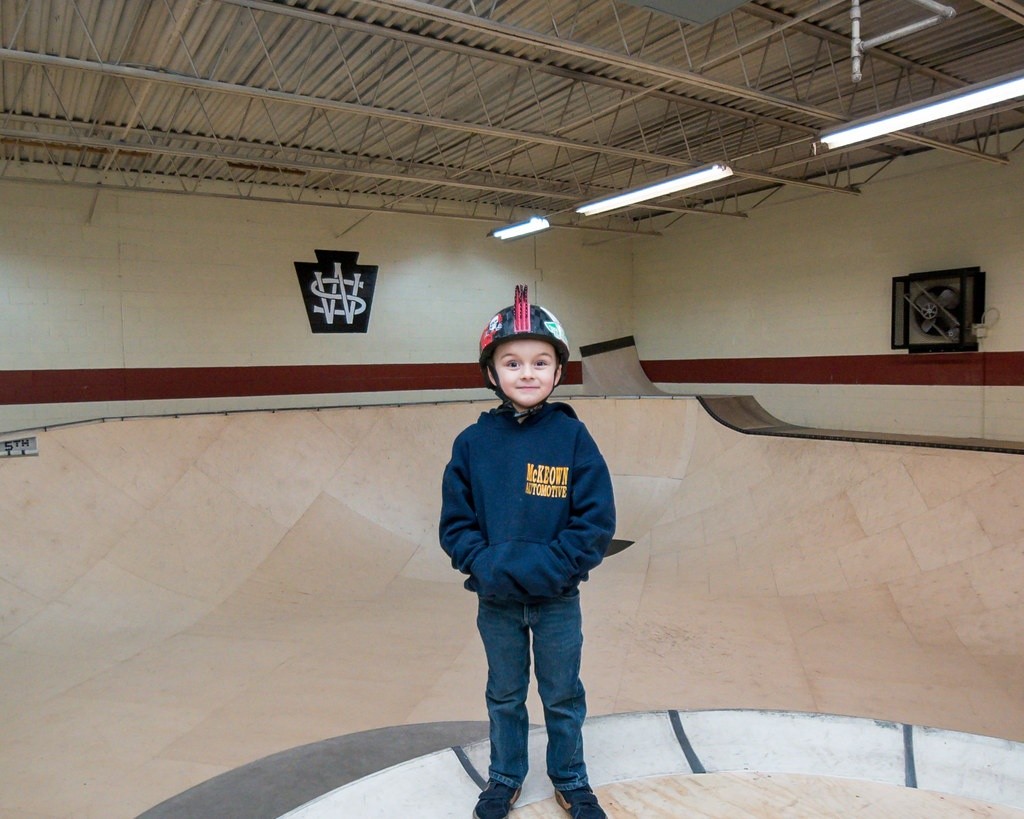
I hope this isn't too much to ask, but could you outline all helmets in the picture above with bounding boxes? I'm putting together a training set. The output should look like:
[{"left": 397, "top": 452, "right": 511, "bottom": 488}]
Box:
[{"left": 476, "top": 303, "right": 571, "bottom": 391}]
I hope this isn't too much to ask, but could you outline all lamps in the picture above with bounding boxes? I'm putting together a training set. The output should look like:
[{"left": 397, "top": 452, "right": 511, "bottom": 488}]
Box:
[
  {"left": 573, "top": 160, "right": 735, "bottom": 216},
  {"left": 484, "top": 213, "right": 551, "bottom": 242},
  {"left": 816, "top": 69, "right": 1024, "bottom": 153}
]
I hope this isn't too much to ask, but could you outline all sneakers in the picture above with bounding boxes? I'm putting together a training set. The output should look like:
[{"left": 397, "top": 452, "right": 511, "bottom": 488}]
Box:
[
  {"left": 471, "top": 778, "right": 523, "bottom": 819},
  {"left": 554, "top": 783, "right": 609, "bottom": 819}
]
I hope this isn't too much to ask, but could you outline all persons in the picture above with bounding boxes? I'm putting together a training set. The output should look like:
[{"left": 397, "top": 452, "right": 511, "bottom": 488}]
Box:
[{"left": 439, "top": 282, "right": 616, "bottom": 819}]
